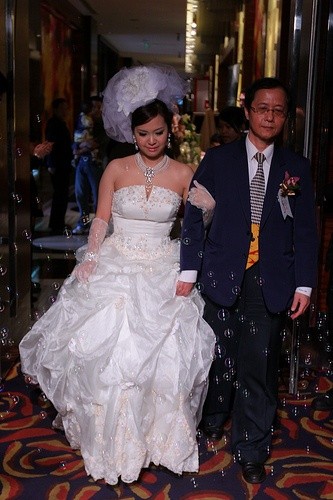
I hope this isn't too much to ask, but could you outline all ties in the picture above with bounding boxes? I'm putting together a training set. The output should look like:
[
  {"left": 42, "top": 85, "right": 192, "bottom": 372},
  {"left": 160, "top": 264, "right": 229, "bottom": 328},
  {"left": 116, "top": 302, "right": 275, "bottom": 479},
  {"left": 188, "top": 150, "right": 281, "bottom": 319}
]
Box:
[{"left": 245, "top": 153, "right": 265, "bottom": 269}]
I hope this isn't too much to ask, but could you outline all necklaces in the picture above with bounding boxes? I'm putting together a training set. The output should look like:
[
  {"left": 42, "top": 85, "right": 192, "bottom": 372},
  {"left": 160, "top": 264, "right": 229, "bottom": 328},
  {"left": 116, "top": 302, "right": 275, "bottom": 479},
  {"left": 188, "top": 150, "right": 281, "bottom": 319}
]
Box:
[{"left": 135, "top": 151, "right": 170, "bottom": 183}]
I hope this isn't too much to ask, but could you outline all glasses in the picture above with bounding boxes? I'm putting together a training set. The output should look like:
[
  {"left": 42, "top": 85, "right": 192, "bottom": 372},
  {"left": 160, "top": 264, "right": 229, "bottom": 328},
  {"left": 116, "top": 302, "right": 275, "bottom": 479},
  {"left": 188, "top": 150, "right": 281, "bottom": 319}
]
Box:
[{"left": 248, "top": 104, "right": 287, "bottom": 117}]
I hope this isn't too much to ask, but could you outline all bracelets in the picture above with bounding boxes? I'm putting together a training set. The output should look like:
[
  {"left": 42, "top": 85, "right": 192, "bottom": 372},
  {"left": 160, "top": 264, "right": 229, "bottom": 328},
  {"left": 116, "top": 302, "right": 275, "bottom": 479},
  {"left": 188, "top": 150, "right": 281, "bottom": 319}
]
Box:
[{"left": 83, "top": 252, "right": 98, "bottom": 262}]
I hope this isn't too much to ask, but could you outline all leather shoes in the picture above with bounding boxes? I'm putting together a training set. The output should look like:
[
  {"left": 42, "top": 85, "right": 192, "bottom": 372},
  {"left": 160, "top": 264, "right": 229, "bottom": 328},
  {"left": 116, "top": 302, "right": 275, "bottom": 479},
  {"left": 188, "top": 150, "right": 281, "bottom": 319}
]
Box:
[
  {"left": 242, "top": 461, "right": 266, "bottom": 483},
  {"left": 204, "top": 418, "right": 225, "bottom": 438}
]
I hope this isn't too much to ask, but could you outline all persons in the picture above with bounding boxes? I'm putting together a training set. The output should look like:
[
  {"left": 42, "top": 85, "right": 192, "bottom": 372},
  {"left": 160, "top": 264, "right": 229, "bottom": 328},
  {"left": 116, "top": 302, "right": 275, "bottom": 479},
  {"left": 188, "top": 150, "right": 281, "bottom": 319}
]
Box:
[
  {"left": 17, "top": 66, "right": 217, "bottom": 486},
  {"left": 176, "top": 77, "right": 321, "bottom": 483},
  {"left": 44, "top": 95, "right": 245, "bottom": 234}
]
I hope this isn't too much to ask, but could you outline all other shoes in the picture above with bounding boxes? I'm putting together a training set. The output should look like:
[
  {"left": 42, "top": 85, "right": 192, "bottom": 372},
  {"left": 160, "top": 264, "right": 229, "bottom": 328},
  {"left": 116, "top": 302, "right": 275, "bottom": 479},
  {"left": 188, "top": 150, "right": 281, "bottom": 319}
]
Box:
[{"left": 72, "top": 225, "right": 90, "bottom": 234}]
